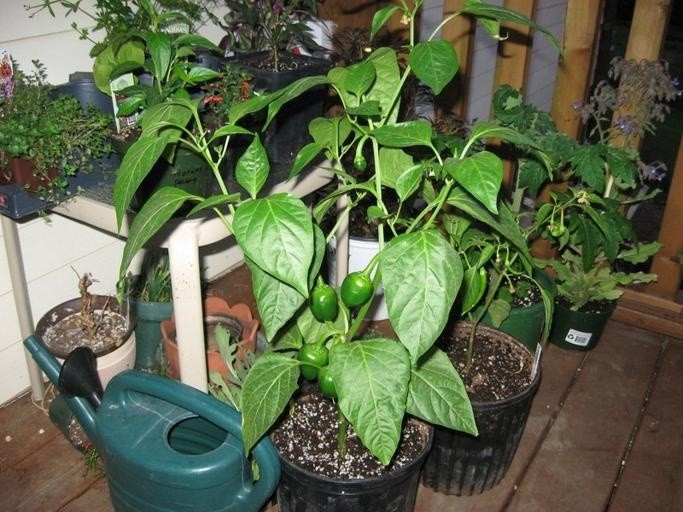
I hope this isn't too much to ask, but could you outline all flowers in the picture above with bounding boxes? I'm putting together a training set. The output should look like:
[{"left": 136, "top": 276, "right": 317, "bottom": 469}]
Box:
[
  {"left": 0, "top": 49, "right": 119, "bottom": 229},
  {"left": 572, "top": 56, "right": 683, "bottom": 185}
]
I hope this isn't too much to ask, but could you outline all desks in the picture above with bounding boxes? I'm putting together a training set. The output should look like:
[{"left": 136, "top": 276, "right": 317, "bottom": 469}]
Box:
[{"left": 61, "top": 150, "right": 351, "bottom": 396}]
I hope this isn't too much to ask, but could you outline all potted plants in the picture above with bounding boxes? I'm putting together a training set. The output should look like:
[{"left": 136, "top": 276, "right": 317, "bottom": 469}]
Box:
[
  {"left": 468, "top": 86, "right": 640, "bottom": 346},
  {"left": 549, "top": 169, "right": 661, "bottom": 351},
  {"left": 40, "top": 3, "right": 332, "bottom": 162},
  {"left": 116, "top": 261, "right": 170, "bottom": 366},
  {"left": 113, "top": 5, "right": 567, "bottom": 510},
  {"left": 418, "top": 151, "right": 600, "bottom": 495},
  {"left": 36, "top": 270, "right": 135, "bottom": 389}
]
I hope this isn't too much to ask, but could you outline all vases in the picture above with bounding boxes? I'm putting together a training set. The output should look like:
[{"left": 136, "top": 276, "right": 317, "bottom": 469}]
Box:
[
  {"left": 164, "top": 298, "right": 259, "bottom": 383},
  {"left": 3, "top": 153, "right": 60, "bottom": 191}
]
